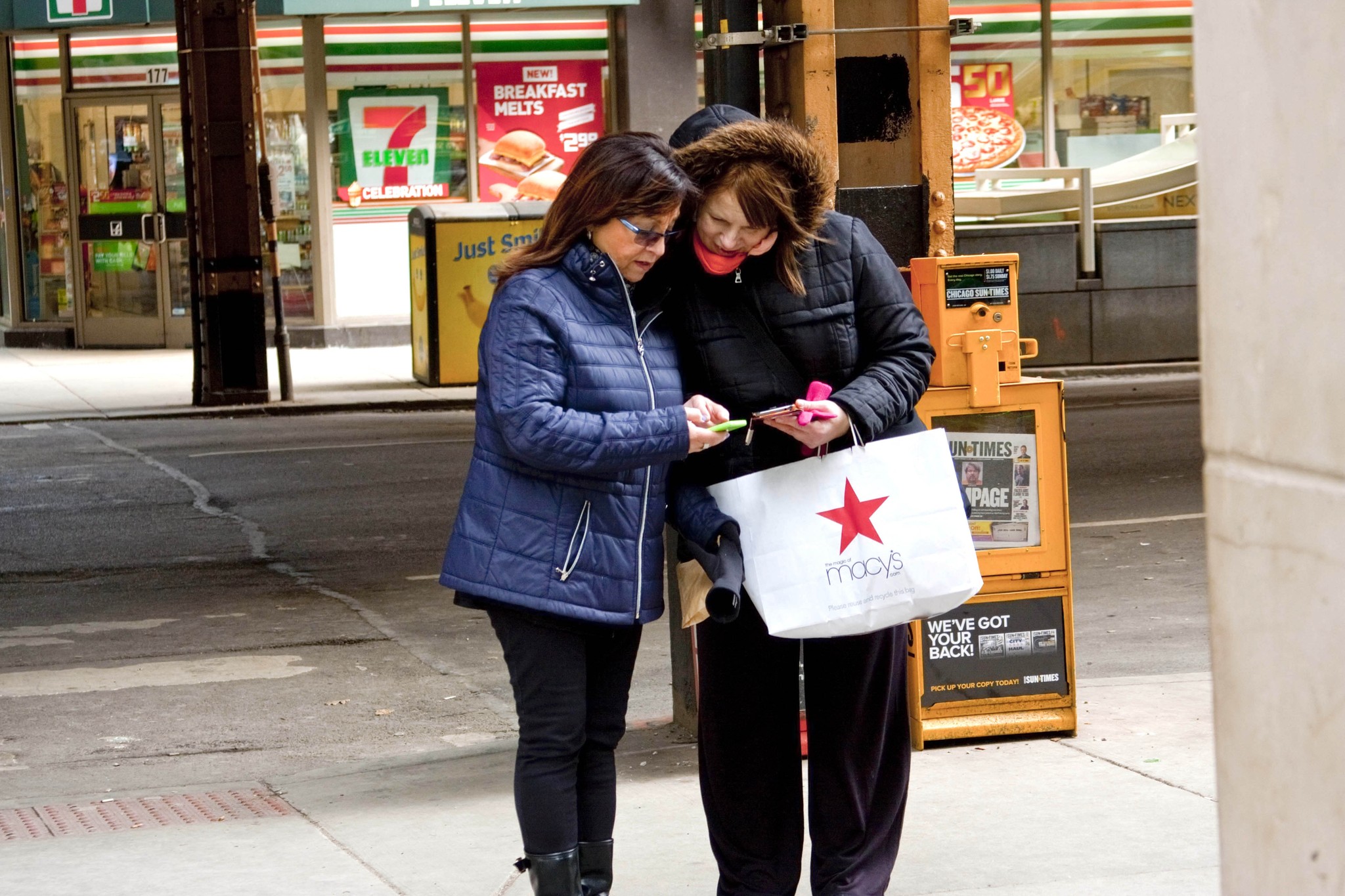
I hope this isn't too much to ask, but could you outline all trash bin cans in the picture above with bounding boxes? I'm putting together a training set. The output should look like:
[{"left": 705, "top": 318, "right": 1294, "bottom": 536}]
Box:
[{"left": 409, "top": 200, "right": 561, "bottom": 388}]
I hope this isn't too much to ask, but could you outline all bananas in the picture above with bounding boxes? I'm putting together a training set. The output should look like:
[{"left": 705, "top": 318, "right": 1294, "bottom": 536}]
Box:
[{"left": 463, "top": 285, "right": 490, "bottom": 328}]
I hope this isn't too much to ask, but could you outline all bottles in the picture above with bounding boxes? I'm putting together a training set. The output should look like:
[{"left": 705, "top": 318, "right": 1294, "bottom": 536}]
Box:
[{"left": 259, "top": 113, "right": 313, "bottom": 252}]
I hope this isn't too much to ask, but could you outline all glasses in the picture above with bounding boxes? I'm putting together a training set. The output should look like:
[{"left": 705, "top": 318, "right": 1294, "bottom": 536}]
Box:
[{"left": 617, "top": 217, "right": 681, "bottom": 247}]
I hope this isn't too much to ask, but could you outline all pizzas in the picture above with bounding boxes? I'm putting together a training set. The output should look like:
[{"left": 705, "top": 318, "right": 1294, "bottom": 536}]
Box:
[{"left": 950, "top": 105, "right": 1022, "bottom": 174}]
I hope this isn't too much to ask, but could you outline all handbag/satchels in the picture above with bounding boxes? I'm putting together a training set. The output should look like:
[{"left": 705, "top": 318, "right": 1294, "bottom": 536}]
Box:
[{"left": 708, "top": 402, "right": 984, "bottom": 639}]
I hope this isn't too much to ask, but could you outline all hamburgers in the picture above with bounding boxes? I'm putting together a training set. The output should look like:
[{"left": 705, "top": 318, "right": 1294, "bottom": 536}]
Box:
[
  {"left": 513, "top": 169, "right": 567, "bottom": 201},
  {"left": 490, "top": 129, "right": 548, "bottom": 172}
]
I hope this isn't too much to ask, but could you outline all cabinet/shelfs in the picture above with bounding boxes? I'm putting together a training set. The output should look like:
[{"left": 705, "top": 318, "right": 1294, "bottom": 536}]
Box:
[{"left": 27, "top": 104, "right": 478, "bottom": 319}]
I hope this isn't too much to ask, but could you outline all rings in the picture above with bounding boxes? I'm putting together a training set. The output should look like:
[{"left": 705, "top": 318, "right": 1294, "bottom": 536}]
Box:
[{"left": 701, "top": 443, "right": 710, "bottom": 449}]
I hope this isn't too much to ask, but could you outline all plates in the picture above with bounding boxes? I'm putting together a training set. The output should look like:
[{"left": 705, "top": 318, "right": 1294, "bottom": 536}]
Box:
[
  {"left": 478, "top": 146, "right": 555, "bottom": 176},
  {"left": 952, "top": 118, "right": 1027, "bottom": 177}
]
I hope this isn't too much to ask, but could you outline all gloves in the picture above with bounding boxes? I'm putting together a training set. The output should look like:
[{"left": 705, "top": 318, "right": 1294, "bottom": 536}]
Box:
[{"left": 686, "top": 518, "right": 745, "bottom": 622}]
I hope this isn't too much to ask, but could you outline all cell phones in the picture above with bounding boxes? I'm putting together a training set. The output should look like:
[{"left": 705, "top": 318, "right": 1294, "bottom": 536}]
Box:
[
  {"left": 708, "top": 419, "right": 747, "bottom": 432},
  {"left": 752, "top": 405, "right": 797, "bottom": 418}
]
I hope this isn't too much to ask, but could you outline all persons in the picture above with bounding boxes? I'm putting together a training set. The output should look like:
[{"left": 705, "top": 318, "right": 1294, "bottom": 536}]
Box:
[
  {"left": 962, "top": 462, "right": 982, "bottom": 484},
  {"left": 647, "top": 103, "right": 972, "bottom": 896},
  {"left": 436, "top": 132, "right": 730, "bottom": 896},
  {"left": 1020, "top": 500, "right": 1028, "bottom": 509},
  {"left": 1017, "top": 445, "right": 1030, "bottom": 458},
  {"left": 1015, "top": 465, "right": 1029, "bottom": 486}
]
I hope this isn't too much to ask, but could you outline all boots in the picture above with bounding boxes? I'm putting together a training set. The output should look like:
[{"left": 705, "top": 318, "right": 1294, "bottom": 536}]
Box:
[
  {"left": 513, "top": 843, "right": 582, "bottom": 896},
  {"left": 577, "top": 838, "right": 614, "bottom": 896}
]
